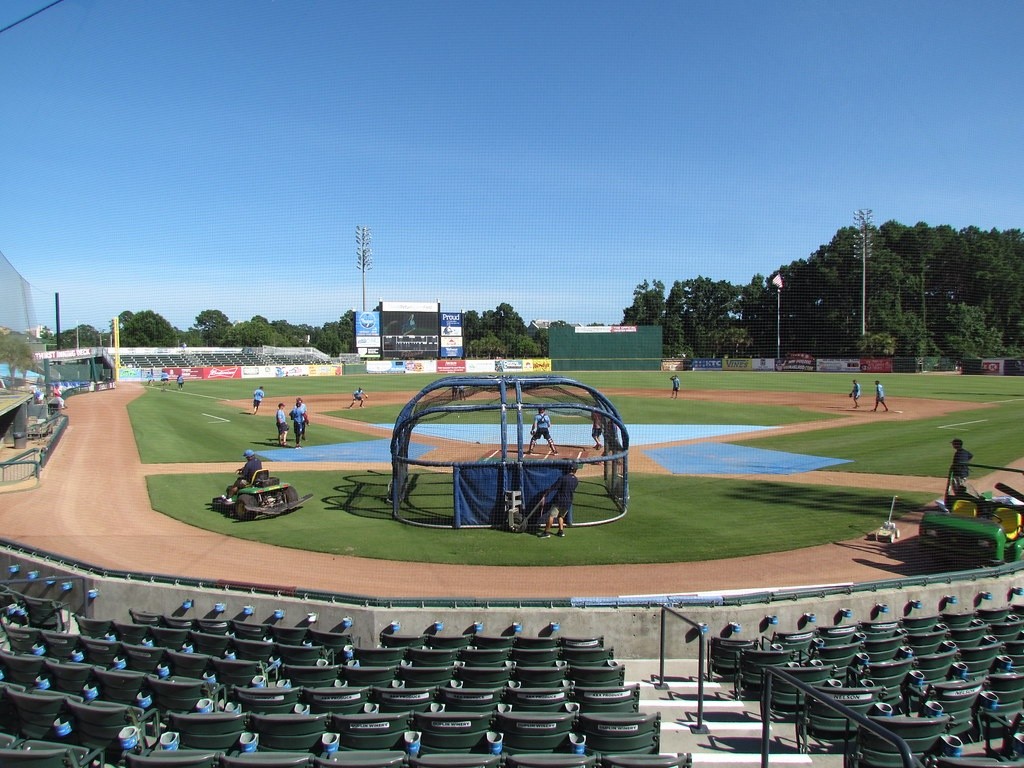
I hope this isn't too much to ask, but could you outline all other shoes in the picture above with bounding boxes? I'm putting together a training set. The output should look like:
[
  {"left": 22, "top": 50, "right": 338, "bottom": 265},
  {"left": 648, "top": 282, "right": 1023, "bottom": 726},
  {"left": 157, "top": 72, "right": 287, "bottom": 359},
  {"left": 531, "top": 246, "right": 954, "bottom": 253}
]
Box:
[
  {"left": 224, "top": 498, "right": 232, "bottom": 503},
  {"left": 295, "top": 446, "right": 302, "bottom": 449},
  {"left": 593, "top": 444, "right": 603, "bottom": 450},
  {"left": 554, "top": 451, "right": 560, "bottom": 456},
  {"left": 527, "top": 451, "right": 532, "bottom": 455},
  {"left": 279, "top": 442, "right": 288, "bottom": 447}
]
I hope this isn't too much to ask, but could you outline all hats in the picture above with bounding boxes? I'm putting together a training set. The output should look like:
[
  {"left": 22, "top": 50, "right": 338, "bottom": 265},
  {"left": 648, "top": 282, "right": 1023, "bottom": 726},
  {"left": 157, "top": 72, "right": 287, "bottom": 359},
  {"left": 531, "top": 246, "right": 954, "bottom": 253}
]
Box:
[
  {"left": 538, "top": 407, "right": 544, "bottom": 411},
  {"left": 243, "top": 450, "right": 254, "bottom": 457},
  {"left": 279, "top": 403, "right": 285, "bottom": 407},
  {"left": 950, "top": 439, "right": 962, "bottom": 445},
  {"left": 296, "top": 403, "right": 301, "bottom": 406},
  {"left": 569, "top": 463, "right": 578, "bottom": 471}
]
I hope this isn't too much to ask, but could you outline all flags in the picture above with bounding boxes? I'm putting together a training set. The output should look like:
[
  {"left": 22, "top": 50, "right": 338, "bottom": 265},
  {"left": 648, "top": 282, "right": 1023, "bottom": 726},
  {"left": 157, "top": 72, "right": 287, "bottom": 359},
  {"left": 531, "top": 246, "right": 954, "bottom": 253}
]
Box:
[{"left": 773, "top": 273, "right": 783, "bottom": 288}]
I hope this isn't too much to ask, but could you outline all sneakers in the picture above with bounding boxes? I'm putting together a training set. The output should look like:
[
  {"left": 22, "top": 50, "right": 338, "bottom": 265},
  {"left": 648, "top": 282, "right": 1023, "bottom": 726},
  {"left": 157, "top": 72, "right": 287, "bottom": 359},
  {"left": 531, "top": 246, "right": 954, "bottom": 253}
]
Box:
[
  {"left": 538, "top": 531, "right": 551, "bottom": 539},
  {"left": 556, "top": 530, "right": 566, "bottom": 537}
]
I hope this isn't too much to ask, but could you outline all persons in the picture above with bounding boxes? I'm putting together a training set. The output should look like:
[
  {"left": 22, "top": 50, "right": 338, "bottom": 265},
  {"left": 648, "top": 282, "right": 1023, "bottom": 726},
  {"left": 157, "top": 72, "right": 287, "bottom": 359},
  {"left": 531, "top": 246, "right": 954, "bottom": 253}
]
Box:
[
  {"left": 950, "top": 439, "right": 974, "bottom": 495},
  {"left": 848, "top": 379, "right": 860, "bottom": 408},
  {"left": 183, "top": 342, "right": 187, "bottom": 350},
  {"left": 145, "top": 372, "right": 154, "bottom": 387},
  {"left": 161, "top": 370, "right": 171, "bottom": 391},
  {"left": 870, "top": 380, "right": 889, "bottom": 412},
  {"left": 348, "top": 387, "right": 368, "bottom": 409},
  {"left": 536, "top": 464, "right": 579, "bottom": 538},
  {"left": 54, "top": 384, "right": 68, "bottom": 409},
  {"left": 528, "top": 408, "right": 559, "bottom": 456},
  {"left": 276, "top": 402, "right": 291, "bottom": 447},
  {"left": 220, "top": 450, "right": 261, "bottom": 501},
  {"left": 32, "top": 381, "right": 43, "bottom": 404},
  {"left": 670, "top": 375, "right": 680, "bottom": 399},
  {"left": 289, "top": 398, "right": 310, "bottom": 449},
  {"left": 955, "top": 359, "right": 962, "bottom": 374},
  {"left": 591, "top": 411, "right": 603, "bottom": 449},
  {"left": 253, "top": 386, "right": 264, "bottom": 415},
  {"left": 177, "top": 375, "right": 184, "bottom": 391}
]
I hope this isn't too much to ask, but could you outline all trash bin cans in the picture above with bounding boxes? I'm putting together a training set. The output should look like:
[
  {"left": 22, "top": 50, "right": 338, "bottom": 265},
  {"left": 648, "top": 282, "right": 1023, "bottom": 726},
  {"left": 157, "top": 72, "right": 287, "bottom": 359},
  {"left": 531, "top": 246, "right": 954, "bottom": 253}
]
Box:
[{"left": 13, "top": 432, "right": 27, "bottom": 449}]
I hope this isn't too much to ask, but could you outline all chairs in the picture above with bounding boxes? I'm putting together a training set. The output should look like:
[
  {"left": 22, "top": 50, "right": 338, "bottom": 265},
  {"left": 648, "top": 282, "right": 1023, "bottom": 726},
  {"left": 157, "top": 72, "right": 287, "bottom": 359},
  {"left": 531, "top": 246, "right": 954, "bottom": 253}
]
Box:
[
  {"left": 953, "top": 499, "right": 1022, "bottom": 539},
  {"left": 244, "top": 469, "right": 270, "bottom": 488},
  {"left": 0, "top": 583, "right": 692, "bottom": 768},
  {"left": 706, "top": 604, "right": 1024, "bottom": 768}
]
